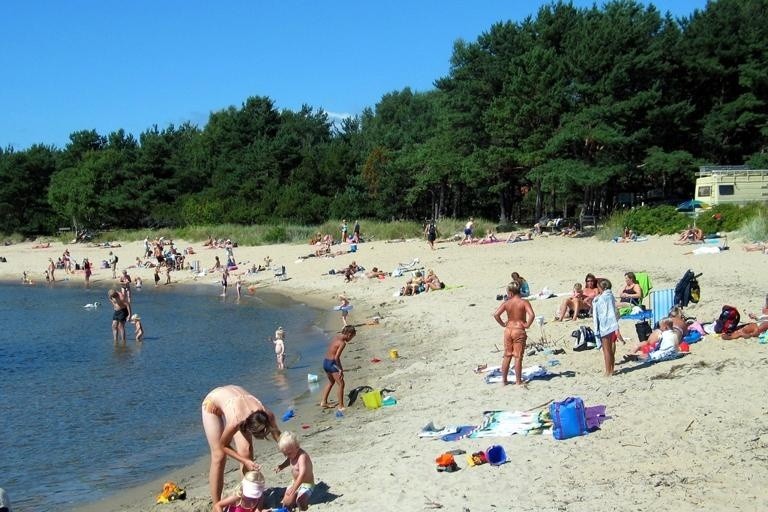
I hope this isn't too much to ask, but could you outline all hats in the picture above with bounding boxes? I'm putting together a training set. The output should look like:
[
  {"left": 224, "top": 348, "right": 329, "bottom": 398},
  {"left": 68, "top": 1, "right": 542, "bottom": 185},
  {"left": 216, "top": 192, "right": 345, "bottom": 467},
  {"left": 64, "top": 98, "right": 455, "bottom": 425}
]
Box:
[{"left": 241, "top": 470, "right": 266, "bottom": 499}]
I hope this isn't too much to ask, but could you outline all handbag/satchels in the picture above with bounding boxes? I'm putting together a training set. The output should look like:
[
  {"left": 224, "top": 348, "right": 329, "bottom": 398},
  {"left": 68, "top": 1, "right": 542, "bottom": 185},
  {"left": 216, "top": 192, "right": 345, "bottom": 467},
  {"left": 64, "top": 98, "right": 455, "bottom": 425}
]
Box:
[
  {"left": 548, "top": 396, "right": 588, "bottom": 439},
  {"left": 712, "top": 304, "right": 740, "bottom": 335},
  {"left": 688, "top": 273, "right": 704, "bottom": 307},
  {"left": 571, "top": 325, "right": 597, "bottom": 352},
  {"left": 635, "top": 316, "right": 653, "bottom": 343}
]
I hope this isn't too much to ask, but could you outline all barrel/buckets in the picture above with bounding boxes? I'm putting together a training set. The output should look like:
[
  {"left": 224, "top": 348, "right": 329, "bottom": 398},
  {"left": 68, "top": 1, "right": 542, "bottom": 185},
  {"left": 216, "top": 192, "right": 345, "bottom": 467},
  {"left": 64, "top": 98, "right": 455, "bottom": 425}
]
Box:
[
  {"left": 535, "top": 316, "right": 544, "bottom": 327},
  {"left": 390, "top": 348, "right": 399, "bottom": 358},
  {"left": 350, "top": 243, "right": 357, "bottom": 251},
  {"left": 486, "top": 445, "right": 507, "bottom": 467}
]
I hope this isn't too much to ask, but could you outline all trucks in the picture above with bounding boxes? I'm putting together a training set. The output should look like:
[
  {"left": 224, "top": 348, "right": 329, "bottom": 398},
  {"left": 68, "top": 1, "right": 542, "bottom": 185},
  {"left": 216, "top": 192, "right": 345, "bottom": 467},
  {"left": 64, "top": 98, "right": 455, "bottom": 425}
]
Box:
[{"left": 694, "top": 168, "right": 768, "bottom": 209}]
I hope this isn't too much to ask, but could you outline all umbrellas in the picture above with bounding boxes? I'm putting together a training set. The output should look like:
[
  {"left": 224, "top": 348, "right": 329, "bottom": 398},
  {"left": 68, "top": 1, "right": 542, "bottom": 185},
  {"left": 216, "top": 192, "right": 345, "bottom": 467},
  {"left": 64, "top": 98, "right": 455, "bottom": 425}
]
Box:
[{"left": 675, "top": 198, "right": 711, "bottom": 227}]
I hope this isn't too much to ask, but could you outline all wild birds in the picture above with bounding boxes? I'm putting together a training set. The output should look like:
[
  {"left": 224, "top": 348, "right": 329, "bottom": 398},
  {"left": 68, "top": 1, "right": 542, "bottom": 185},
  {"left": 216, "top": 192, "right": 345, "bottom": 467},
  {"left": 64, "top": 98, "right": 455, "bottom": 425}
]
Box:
[{"left": 84, "top": 301, "right": 101, "bottom": 309}]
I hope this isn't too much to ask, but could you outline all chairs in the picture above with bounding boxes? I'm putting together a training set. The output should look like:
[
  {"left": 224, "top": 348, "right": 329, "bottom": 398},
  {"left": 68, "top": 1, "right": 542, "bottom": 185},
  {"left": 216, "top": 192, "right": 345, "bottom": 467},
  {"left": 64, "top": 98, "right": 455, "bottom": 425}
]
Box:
[
  {"left": 613, "top": 271, "right": 653, "bottom": 317},
  {"left": 648, "top": 286, "right": 678, "bottom": 330},
  {"left": 409, "top": 270, "right": 426, "bottom": 293}
]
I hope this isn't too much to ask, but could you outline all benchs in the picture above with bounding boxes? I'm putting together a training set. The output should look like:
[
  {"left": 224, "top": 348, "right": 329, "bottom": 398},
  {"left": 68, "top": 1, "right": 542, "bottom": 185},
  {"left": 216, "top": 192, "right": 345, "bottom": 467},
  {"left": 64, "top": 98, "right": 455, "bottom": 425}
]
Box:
[{"left": 539, "top": 218, "right": 560, "bottom": 233}]
